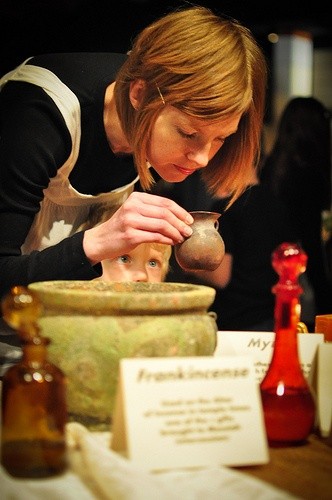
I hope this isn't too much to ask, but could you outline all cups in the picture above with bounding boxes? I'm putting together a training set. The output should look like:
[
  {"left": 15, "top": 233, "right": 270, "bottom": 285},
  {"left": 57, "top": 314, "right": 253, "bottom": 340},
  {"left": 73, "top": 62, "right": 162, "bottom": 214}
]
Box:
[{"left": 173, "top": 212, "right": 225, "bottom": 271}]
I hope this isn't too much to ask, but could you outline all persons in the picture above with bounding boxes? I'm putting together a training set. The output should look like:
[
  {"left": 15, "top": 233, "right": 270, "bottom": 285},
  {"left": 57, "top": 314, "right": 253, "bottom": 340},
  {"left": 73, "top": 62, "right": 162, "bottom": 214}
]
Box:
[
  {"left": 71, "top": 195, "right": 173, "bottom": 286},
  {"left": 0, "top": 10, "right": 266, "bottom": 288}
]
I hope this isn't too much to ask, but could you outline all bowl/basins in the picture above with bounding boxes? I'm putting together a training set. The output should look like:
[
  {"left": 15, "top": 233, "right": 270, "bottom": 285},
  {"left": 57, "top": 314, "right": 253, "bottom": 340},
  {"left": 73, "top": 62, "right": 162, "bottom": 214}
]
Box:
[{"left": 28, "top": 281, "right": 216, "bottom": 418}]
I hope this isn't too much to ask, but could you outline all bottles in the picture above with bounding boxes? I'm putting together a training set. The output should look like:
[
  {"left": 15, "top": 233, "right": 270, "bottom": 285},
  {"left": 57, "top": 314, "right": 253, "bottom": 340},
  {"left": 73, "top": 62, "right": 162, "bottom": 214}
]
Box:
[
  {"left": 0, "top": 286, "right": 67, "bottom": 478},
  {"left": 259, "top": 243, "right": 315, "bottom": 444}
]
